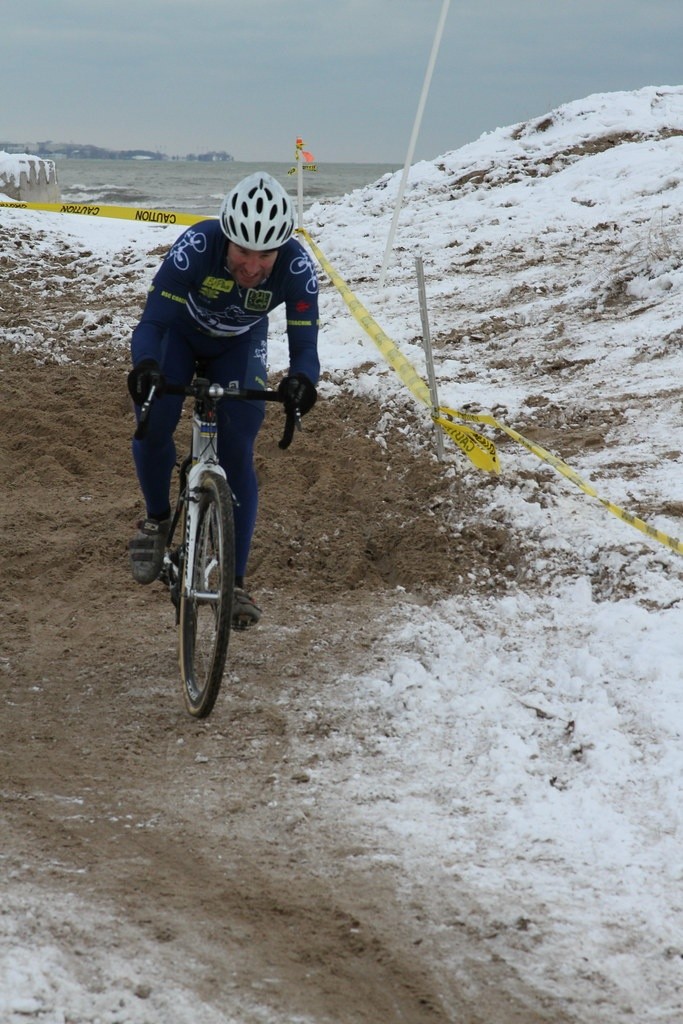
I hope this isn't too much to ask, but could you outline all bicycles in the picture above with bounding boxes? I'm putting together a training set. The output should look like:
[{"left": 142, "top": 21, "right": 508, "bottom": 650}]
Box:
[{"left": 133, "top": 369, "right": 306, "bottom": 718}]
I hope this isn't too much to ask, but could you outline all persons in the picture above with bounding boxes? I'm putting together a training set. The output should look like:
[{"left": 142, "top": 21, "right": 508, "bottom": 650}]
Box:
[{"left": 125, "top": 172, "right": 320, "bottom": 626}]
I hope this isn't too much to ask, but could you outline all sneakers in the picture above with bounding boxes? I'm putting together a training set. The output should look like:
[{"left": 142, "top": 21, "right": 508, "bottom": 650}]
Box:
[
  {"left": 129, "top": 517, "right": 174, "bottom": 585},
  {"left": 233, "top": 587, "right": 259, "bottom": 631}
]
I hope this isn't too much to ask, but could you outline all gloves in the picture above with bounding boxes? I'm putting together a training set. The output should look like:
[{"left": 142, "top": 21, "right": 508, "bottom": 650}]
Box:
[
  {"left": 276, "top": 371, "right": 318, "bottom": 416},
  {"left": 127, "top": 359, "right": 172, "bottom": 406}
]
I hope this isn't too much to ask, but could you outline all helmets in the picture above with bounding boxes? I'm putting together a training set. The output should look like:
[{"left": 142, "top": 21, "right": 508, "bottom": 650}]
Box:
[{"left": 219, "top": 169, "right": 293, "bottom": 251}]
